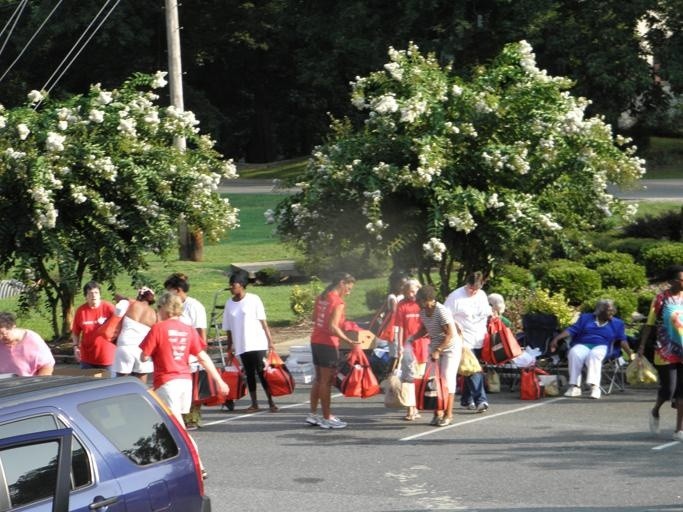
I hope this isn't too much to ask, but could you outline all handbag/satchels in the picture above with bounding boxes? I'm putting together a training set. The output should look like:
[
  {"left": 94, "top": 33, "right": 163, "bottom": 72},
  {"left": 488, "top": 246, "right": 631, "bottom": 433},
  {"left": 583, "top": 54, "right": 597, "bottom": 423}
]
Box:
[
  {"left": 220, "top": 349, "right": 248, "bottom": 400},
  {"left": 495, "top": 361, "right": 522, "bottom": 393},
  {"left": 414, "top": 359, "right": 449, "bottom": 410},
  {"left": 537, "top": 365, "right": 567, "bottom": 398},
  {"left": 94, "top": 313, "right": 124, "bottom": 345},
  {"left": 193, "top": 366, "right": 226, "bottom": 406},
  {"left": 520, "top": 365, "right": 550, "bottom": 400},
  {"left": 368, "top": 301, "right": 396, "bottom": 344},
  {"left": 481, "top": 317, "right": 522, "bottom": 365},
  {"left": 262, "top": 346, "right": 295, "bottom": 396},
  {"left": 332, "top": 343, "right": 381, "bottom": 399},
  {"left": 384, "top": 358, "right": 416, "bottom": 408},
  {"left": 485, "top": 368, "right": 501, "bottom": 393}
]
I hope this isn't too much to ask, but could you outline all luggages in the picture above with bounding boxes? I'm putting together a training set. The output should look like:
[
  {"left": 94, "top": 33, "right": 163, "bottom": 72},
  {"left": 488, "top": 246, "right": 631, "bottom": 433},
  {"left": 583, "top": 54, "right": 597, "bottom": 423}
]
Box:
[{"left": 362, "top": 338, "right": 395, "bottom": 384}]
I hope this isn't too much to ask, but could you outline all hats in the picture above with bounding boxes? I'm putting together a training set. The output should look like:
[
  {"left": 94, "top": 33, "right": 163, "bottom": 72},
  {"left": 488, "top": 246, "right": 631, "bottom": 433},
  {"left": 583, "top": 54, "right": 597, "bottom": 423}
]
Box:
[{"left": 230, "top": 269, "right": 250, "bottom": 287}]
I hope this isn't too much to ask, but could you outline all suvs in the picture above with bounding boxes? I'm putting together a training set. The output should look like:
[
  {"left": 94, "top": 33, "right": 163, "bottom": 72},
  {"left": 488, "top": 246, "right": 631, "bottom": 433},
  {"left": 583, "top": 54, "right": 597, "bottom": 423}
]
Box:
[{"left": 0, "top": 373, "right": 210, "bottom": 512}]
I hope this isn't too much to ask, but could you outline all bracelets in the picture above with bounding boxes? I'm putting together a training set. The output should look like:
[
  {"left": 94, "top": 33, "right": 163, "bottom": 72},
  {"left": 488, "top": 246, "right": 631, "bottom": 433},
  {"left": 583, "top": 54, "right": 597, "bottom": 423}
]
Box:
[{"left": 640, "top": 346, "right": 644, "bottom": 349}]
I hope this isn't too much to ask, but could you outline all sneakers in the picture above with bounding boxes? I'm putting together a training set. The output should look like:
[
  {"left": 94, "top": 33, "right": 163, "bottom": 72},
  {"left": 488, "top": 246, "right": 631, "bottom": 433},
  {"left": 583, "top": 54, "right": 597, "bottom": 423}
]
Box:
[
  {"left": 402, "top": 412, "right": 454, "bottom": 427},
  {"left": 476, "top": 401, "right": 488, "bottom": 413},
  {"left": 671, "top": 430, "right": 683, "bottom": 441},
  {"left": 185, "top": 421, "right": 199, "bottom": 431},
  {"left": 468, "top": 402, "right": 476, "bottom": 410},
  {"left": 270, "top": 402, "right": 279, "bottom": 412},
  {"left": 305, "top": 413, "right": 325, "bottom": 425},
  {"left": 648, "top": 408, "right": 661, "bottom": 435},
  {"left": 245, "top": 404, "right": 260, "bottom": 413},
  {"left": 564, "top": 386, "right": 582, "bottom": 397},
  {"left": 319, "top": 417, "right": 348, "bottom": 429},
  {"left": 589, "top": 386, "right": 601, "bottom": 399}
]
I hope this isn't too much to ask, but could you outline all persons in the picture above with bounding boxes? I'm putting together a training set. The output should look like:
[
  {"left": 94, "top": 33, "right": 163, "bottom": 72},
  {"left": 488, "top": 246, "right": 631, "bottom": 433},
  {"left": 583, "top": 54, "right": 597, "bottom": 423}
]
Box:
[
  {"left": 1, "top": 312, "right": 55, "bottom": 380},
  {"left": 139, "top": 293, "right": 229, "bottom": 478},
  {"left": 367, "top": 271, "right": 516, "bottom": 428},
  {"left": 222, "top": 270, "right": 278, "bottom": 413},
  {"left": 638, "top": 268, "right": 683, "bottom": 441},
  {"left": 102, "top": 288, "right": 157, "bottom": 385},
  {"left": 550, "top": 299, "right": 634, "bottom": 399},
  {"left": 73, "top": 281, "right": 116, "bottom": 379},
  {"left": 163, "top": 273, "right": 208, "bottom": 402},
  {"left": 306, "top": 271, "right": 361, "bottom": 429}
]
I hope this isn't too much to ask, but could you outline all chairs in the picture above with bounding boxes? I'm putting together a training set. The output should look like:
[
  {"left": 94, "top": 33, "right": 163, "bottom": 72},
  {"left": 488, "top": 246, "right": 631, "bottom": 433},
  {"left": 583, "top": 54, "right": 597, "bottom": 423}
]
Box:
[{"left": 479, "top": 314, "right": 626, "bottom": 397}]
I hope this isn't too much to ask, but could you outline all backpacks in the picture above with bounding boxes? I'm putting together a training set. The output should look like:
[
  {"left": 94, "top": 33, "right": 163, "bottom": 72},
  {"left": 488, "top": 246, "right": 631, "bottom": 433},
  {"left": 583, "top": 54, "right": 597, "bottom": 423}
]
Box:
[{"left": 520, "top": 309, "right": 568, "bottom": 359}]
